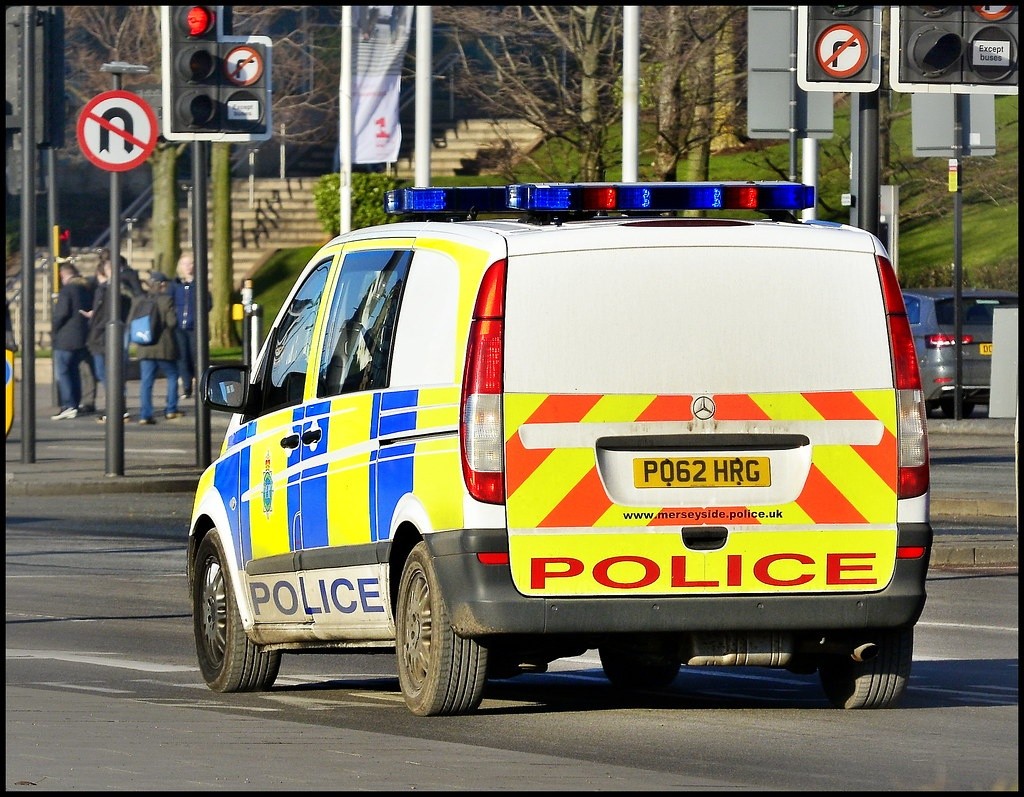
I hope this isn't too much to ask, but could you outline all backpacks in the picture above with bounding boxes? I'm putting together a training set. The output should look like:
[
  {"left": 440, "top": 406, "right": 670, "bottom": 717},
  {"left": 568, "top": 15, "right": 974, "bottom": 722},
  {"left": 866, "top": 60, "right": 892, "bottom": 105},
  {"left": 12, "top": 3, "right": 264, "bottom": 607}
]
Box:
[{"left": 128, "top": 293, "right": 162, "bottom": 344}]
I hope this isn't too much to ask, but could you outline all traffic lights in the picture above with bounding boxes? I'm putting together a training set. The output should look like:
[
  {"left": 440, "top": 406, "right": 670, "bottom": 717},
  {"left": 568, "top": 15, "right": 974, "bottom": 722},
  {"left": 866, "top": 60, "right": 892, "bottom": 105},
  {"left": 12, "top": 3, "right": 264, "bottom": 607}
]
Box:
[
  {"left": 900, "top": 2, "right": 964, "bottom": 83},
  {"left": 174, "top": 9, "right": 220, "bottom": 134}
]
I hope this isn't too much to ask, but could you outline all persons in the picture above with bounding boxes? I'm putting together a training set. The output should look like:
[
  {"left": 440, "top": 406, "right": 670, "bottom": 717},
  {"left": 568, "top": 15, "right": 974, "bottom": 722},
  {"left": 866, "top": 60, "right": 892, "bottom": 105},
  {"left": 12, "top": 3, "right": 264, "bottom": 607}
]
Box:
[
  {"left": 49, "top": 263, "right": 91, "bottom": 422},
  {"left": 124, "top": 271, "right": 185, "bottom": 425},
  {"left": 80, "top": 248, "right": 143, "bottom": 424},
  {"left": 169, "top": 250, "right": 213, "bottom": 400}
]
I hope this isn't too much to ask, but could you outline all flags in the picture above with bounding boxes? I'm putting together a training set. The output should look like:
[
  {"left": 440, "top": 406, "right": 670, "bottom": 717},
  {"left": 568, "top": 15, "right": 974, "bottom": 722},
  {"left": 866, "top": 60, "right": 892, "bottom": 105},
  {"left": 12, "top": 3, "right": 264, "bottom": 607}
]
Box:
[{"left": 346, "top": 6, "right": 413, "bottom": 163}]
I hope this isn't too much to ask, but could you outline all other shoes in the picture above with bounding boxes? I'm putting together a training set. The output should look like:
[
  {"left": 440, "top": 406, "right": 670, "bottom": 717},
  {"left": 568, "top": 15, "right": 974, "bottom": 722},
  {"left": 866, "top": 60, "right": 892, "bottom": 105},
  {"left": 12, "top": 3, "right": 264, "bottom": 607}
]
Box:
[
  {"left": 180, "top": 393, "right": 190, "bottom": 399},
  {"left": 140, "top": 417, "right": 156, "bottom": 425},
  {"left": 50, "top": 408, "right": 77, "bottom": 421},
  {"left": 95, "top": 413, "right": 130, "bottom": 423},
  {"left": 78, "top": 404, "right": 93, "bottom": 412},
  {"left": 165, "top": 412, "right": 184, "bottom": 418}
]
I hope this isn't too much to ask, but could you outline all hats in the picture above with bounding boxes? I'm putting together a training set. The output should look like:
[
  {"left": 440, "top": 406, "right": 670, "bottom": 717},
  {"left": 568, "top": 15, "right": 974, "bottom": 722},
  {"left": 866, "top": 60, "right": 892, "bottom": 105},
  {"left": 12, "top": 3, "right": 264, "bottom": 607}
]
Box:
[{"left": 150, "top": 271, "right": 173, "bottom": 282}]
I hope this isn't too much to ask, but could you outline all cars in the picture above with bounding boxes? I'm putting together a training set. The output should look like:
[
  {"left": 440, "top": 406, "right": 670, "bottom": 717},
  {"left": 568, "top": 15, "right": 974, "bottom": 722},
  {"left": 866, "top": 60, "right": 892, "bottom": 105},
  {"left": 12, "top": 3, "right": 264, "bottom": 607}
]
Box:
[{"left": 903, "top": 287, "right": 1019, "bottom": 419}]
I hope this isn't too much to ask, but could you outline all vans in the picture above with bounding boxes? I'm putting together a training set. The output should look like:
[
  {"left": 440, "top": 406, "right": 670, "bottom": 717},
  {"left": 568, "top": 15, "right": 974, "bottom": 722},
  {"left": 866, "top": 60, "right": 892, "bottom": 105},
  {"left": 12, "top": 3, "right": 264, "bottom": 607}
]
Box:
[{"left": 185, "top": 171, "right": 932, "bottom": 717}]
